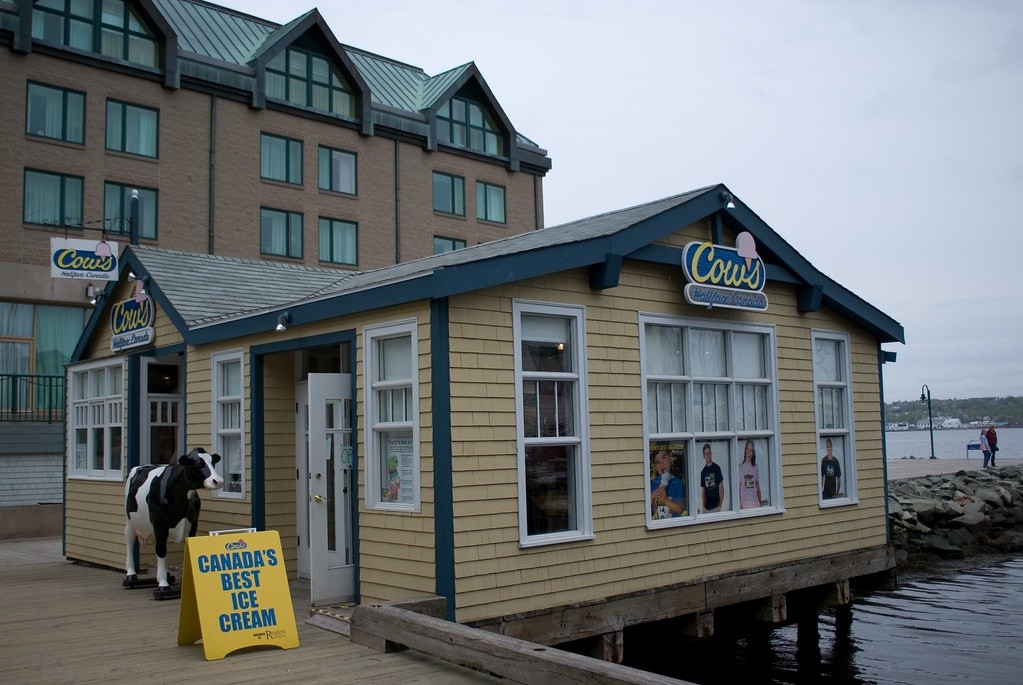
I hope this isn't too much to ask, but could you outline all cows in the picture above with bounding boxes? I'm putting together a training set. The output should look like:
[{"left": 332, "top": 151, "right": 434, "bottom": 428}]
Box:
[{"left": 124, "top": 446, "right": 224, "bottom": 591}]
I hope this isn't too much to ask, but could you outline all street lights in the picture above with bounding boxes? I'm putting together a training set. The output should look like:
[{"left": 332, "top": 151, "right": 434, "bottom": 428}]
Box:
[{"left": 920, "top": 384, "right": 936, "bottom": 459}]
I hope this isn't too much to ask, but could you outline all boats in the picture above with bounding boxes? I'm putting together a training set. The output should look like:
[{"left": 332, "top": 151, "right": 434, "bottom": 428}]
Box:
[{"left": 885, "top": 412, "right": 998, "bottom": 432}]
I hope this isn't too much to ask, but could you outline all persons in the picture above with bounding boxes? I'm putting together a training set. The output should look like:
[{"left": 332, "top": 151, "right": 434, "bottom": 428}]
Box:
[
  {"left": 738, "top": 440, "right": 763, "bottom": 510},
  {"left": 700, "top": 443, "right": 725, "bottom": 513},
  {"left": 821, "top": 438, "right": 842, "bottom": 500},
  {"left": 980, "top": 425, "right": 999, "bottom": 468},
  {"left": 651, "top": 449, "right": 684, "bottom": 520}
]
